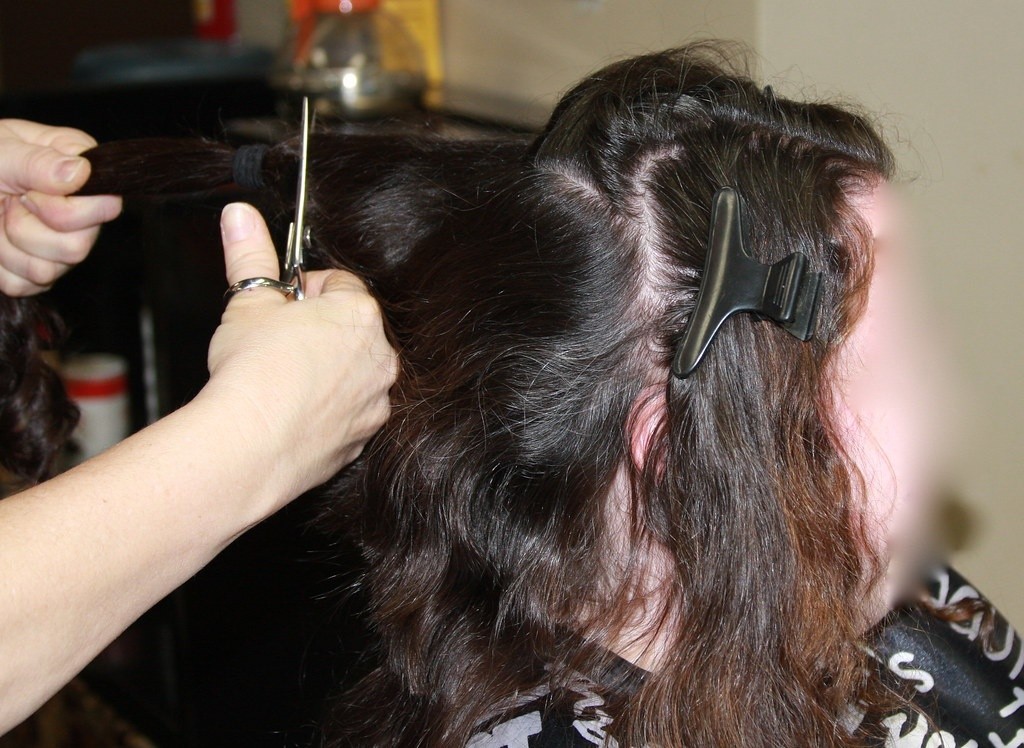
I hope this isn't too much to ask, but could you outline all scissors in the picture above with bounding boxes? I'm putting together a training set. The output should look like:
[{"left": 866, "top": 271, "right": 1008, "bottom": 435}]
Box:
[{"left": 220, "top": 90, "right": 318, "bottom": 309}]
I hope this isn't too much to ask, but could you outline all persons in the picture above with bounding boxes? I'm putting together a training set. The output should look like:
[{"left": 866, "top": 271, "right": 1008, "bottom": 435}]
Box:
[
  {"left": 0, "top": 119, "right": 401, "bottom": 740},
  {"left": 0, "top": 48, "right": 894, "bottom": 748}
]
